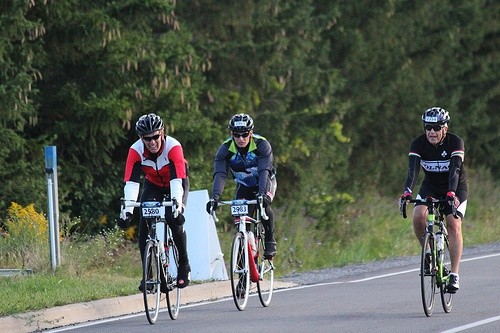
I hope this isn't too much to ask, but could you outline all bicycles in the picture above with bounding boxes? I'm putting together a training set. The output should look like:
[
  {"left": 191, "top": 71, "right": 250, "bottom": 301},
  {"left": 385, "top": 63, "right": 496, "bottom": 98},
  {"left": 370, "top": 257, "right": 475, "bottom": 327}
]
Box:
[
  {"left": 402, "top": 194, "right": 454, "bottom": 318},
  {"left": 120, "top": 197, "right": 180, "bottom": 326},
  {"left": 208, "top": 197, "right": 274, "bottom": 312}
]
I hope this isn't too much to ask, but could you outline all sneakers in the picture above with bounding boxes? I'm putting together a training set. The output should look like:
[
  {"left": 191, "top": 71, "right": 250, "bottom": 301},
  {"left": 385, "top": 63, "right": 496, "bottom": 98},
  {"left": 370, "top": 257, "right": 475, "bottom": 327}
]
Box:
[
  {"left": 138, "top": 276, "right": 154, "bottom": 293},
  {"left": 176, "top": 264, "right": 192, "bottom": 288},
  {"left": 447, "top": 273, "right": 460, "bottom": 290},
  {"left": 236, "top": 273, "right": 247, "bottom": 298},
  {"left": 264, "top": 238, "right": 278, "bottom": 257},
  {"left": 424, "top": 247, "right": 432, "bottom": 270}
]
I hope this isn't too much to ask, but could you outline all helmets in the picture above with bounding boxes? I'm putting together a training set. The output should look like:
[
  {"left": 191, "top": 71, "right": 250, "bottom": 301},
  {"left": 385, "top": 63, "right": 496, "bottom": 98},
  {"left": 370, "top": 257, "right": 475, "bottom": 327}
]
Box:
[
  {"left": 227, "top": 113, "right": 254, "bottom": 133},
  {"left": 421, "top": 106, "right": 451, "bottom": 126},
  {"left": 134, "top": 113, "right": 165, "bottom": 136}
]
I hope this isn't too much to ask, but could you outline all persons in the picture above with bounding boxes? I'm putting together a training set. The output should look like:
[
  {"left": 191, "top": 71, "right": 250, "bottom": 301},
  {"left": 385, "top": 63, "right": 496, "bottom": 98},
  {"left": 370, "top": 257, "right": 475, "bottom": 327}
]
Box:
[
  {"left": 206, "top": 114, "right": 277, "bottom": 298},
  {"left": 398, "top": 107, "right": 468, "bottom": 290},
  {"left": 118, "top": 113, "right": 192, "bottom": 292}
]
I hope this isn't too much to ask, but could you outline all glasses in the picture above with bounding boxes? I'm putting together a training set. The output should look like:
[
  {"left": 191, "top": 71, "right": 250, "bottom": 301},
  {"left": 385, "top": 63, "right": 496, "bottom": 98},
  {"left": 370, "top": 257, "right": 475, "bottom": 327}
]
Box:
[
  {"left": 141, "top": 131, "right": 162, "bottom": 141},
  {"left": 425, "top": 124, "right": 445, "bottom": 132},
  {"left": 232, "top": 131, "right": 250, "bottom": 138}
]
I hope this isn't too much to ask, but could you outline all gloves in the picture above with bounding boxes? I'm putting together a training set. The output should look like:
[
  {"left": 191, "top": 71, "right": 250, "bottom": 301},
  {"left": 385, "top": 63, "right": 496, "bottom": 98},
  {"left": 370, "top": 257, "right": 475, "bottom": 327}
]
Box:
[
  {"left": 117, "top": 211, "right": 135, "bottom": 229},
  {"left": 170, "top": 210, "right": 185, "bottom": 226},
  {"left": 206, "top": 198, "right": 218, "bottom": 214},
  {"left": 256, "top": 194, "right": 267, "bottom": 209}
]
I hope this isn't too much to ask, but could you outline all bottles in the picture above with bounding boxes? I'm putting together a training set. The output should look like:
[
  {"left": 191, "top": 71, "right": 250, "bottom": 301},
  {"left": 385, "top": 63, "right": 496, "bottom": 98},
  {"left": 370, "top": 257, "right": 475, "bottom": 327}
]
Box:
[
  {"left": 436, "top": 231, "right": 445, "bottom": 251},
  {"left": 157, "top": 240, "right": 166, "bottom": 265},
  {"left": 248, "top": 230, "right": 257, "bottom": 257}
]
